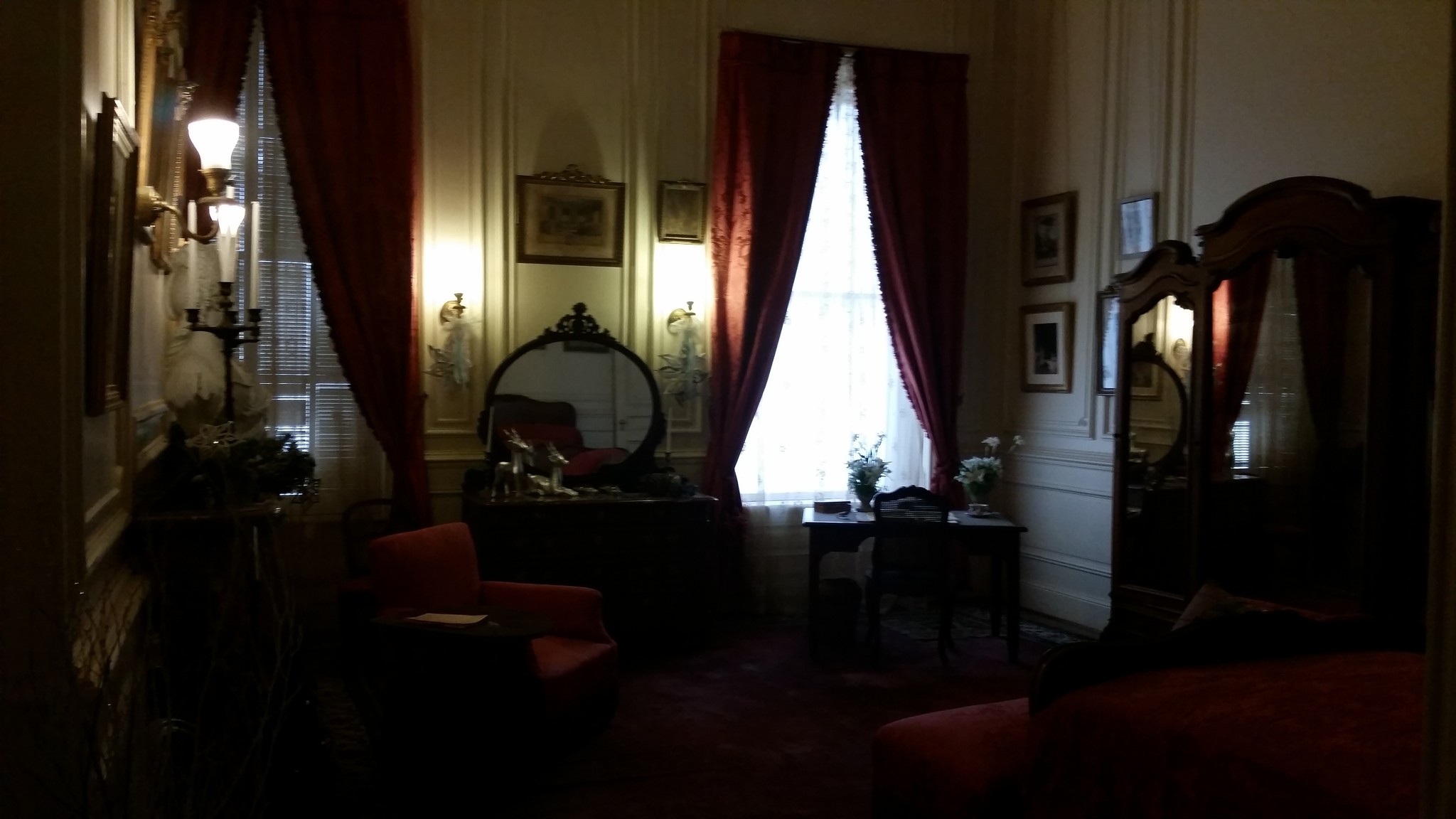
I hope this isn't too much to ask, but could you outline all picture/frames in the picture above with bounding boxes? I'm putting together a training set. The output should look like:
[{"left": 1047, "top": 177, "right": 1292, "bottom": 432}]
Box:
[
  {"left": 654, "top": 177, "right": 708, "bottom": 245},
  {"left": 1086, "top": 284, "right": 1163, "bottom": 399},
  {"left": 1016, "top": 188, "right": 1079, "bottom": 289},
  {"left": 84, "top": 91, "right": 138, "bottom": 420},
  {"left": 514, "top": 167, "right": 628, "bottom": 268},
  {"left": 1114, "top": 191, "right": 1159, "bottom": 263},
  {"left": 1017, "top": 301, "right": 1076, "bottom": 395}
]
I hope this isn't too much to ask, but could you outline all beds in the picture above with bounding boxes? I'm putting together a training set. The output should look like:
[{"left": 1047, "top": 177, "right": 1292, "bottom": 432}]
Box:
[
  {"left": 494, "top": 393, "right": 634, "bottom": 474},
  {"left": 869, "top": 582, "right": 1438, "bottom": 819}
]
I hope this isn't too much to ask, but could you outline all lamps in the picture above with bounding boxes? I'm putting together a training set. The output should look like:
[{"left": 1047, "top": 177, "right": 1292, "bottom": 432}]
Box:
[
  {"left": 134, "top": 78, "right": 245, "bottom": 252},
  {"left": 214, "top": 200, "right": 247, "bottom": 242}
]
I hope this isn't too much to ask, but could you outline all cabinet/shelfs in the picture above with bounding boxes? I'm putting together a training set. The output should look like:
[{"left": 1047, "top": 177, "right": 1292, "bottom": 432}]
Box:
[{"left": 457, "top": 463, "right": 731, "bottom": 662}]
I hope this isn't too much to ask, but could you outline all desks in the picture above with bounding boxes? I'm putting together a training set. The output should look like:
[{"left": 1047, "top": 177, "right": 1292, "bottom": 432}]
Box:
[
  {"left": 138, "top": 494, "right": 302, "bottom": 718},
  {"left": 800, "top": 512, "right": 1028, "bottom": 669}
]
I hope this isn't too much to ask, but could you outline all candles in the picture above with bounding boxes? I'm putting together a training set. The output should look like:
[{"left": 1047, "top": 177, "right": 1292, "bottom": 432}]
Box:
[
  {"left": 184, "top": 195, "right": 199, "bottom": 307},
  {"left": 249, "top": 200, "right": 259, "bottom": 307},
  {"left": 218, "top": 173, "right": 238, "bottom": 281}
]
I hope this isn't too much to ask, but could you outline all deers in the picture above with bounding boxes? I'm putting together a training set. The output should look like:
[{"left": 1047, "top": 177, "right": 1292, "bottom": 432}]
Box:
[
  {"left": 486, "top": 426, "right": 535, "bottom": 504},
  {"left": 525, "top": 439, "right": 580, "bottom": 499}
]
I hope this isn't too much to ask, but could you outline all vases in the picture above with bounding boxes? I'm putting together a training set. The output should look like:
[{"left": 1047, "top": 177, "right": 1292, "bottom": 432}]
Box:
[
  {"left": 854, "top": 489, "right": 876, "bottom": 512},
  {"left": 968, "top": 493, "right": 983, "bottom": 504}
]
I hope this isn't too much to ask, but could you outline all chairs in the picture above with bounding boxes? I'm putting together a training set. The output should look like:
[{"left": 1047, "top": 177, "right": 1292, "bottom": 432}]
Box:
[
  {"left": 335, "top": 493, "right": 413, "bottom": 649},
  {"left": 863, "top": 482, "right": 951, "bottom": 661}
]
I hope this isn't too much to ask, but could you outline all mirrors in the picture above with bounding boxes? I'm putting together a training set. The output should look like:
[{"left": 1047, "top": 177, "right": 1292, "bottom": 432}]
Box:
[{"left": 474, "top": 299, "right": 670, "bottom": 488}]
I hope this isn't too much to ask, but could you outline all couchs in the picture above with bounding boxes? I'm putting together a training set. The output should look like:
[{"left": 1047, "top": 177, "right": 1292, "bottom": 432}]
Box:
[{"left": 364, "top": 524, "right": 623, "bottom": 759}]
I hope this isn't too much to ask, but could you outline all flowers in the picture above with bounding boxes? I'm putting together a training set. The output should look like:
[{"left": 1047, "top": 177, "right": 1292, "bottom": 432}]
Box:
[
  {"left": 946, "top": 436, "right": 1006, "bottom": 498},
  {"left": 842, "top": 425, "right": 894, "bottom": 492}
]
